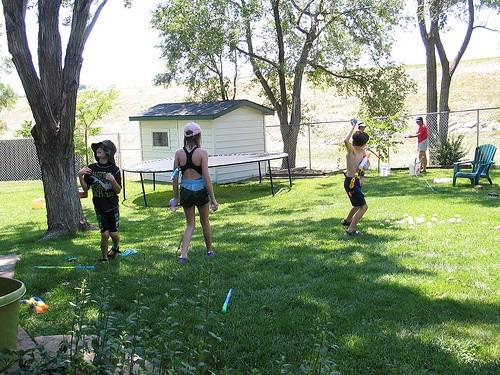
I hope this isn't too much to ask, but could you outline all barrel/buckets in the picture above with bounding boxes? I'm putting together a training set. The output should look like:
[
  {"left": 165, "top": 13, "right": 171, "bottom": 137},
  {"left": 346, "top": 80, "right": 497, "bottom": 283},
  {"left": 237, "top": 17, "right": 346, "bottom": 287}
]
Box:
[
  {"left": 380, "top": 164, "right": 391, "bottom": 177},
  {"left": 0, "top": 277, "right": 26, "bottom": 351},
  {"left": 409, "top": 162, "right": 420, "bottom": 175}
]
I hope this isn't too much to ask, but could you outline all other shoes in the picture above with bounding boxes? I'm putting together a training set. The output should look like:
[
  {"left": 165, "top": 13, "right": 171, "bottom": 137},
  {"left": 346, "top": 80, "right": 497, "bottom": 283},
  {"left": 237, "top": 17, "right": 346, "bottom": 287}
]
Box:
[
  {"left": 344, "top": 230, "right": 364, "bottom": 238},
  {"left": 100, "top": 259, "right": 109, "bottom": 264},
  {"left": 205, "top": 250, "right": 216, "bottom": 257},
  {"left": 107, "top": 245, "right": 121, "bottom": 257},
  {"left": 340, "top": 218, "right": 351, "bottom": 226},
  {"left": 178, "top": 256, "right": 191, "bottom": 263}
]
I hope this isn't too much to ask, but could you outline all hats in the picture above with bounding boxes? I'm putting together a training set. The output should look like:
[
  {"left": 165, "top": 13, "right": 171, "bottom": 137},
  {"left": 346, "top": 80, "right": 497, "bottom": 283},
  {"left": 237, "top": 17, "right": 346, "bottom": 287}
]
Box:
[
  {"left": 91, "top": 140, "right": 117, "bottom": 166},
  {"left": 184, "top": 121, "right": 203, "bottom": 137},
  {"left": 416, "top": 117, "right": 423, "bottom": 122},
  {"left": 358, "top": 122, "right": 367, "bottom": 127}
]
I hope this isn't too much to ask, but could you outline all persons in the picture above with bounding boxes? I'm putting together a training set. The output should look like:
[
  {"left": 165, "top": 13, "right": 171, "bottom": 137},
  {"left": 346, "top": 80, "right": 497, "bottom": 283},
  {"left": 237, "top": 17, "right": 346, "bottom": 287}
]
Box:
[
  {"left": 78, "top": 140, "right": 122, "bottom": 263},
  {"left": 173, "top": 122, "right": 218, "bottom": 262},
  {"left": 341, "top": 118, "right": 370, "bottom": 236},
  {"left": 408, "top": 117, "right": 428, "bottom": 174}
]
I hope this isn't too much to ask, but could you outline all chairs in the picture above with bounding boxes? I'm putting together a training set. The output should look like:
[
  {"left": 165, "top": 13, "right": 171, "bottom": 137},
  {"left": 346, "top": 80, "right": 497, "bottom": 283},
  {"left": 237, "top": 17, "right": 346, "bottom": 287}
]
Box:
[{"left": 452, "top": 144, "right": 497, "bottom": 187}]
